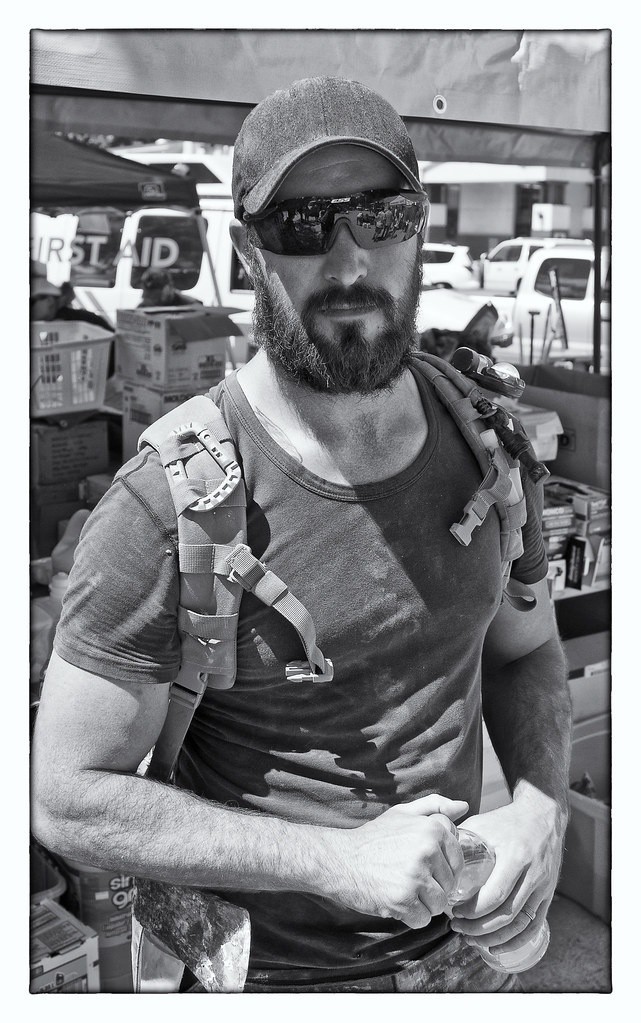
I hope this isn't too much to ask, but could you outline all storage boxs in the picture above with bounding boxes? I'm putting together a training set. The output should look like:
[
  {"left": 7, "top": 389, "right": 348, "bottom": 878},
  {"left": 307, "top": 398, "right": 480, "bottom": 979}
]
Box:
[
  {"left": 30, "top": 319, "right": 115, "bottom": 417},
  {"left": 28, "top": 898, "right": 100, "bottom": 994},
  {"left": 115, "top": 306, "right": 226, "bottom": 427},
  {"left": 30, "top": 420, "right": 110, "bottom": 560},
  {"left": 517, "top": 364, "right": 611, "bottom": 492},
  {"left": 559, "top": 714, "right": 611, "bottom": 926}
]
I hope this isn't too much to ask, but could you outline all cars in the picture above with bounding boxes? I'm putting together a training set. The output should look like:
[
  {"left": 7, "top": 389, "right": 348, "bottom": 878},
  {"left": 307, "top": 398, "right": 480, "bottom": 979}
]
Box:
[{"left": 416, "top": 243, "right": 483, "bottom": 291}]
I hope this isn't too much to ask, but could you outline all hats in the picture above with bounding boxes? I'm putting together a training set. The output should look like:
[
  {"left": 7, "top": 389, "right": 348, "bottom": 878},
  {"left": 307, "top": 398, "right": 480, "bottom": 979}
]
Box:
[{"left": 233, "top": 76, "right": 423, "bottom": 221}]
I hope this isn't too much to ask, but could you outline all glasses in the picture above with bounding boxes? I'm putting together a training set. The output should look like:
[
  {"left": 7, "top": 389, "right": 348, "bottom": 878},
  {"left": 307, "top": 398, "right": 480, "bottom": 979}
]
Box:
[{"left": 243, "top": 192, "right": 428, "bottom": 256}]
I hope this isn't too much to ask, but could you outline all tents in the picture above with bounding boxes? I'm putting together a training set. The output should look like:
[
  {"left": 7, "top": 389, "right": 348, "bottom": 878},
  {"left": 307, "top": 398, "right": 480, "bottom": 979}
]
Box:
[
  {"left": 30, "top": 28, "right": 611, "bottom": 374},
  {"left": 30, "top": 133, "right": 236, "bottom": 371},
  {"left": 390, "top": 195, "right": 415, "bottom": 209}
]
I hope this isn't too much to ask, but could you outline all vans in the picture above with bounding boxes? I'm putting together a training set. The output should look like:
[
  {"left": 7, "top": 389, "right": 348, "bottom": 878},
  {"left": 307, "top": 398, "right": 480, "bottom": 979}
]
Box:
[{"left": 511, "top": 246, "right": 610, "bottom": 370}]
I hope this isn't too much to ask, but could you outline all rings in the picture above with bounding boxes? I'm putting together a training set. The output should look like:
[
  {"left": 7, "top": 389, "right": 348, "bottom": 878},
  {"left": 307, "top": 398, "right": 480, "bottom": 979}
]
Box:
[{"left": 522, "top": 905, "right": 536, "bottom": 920}]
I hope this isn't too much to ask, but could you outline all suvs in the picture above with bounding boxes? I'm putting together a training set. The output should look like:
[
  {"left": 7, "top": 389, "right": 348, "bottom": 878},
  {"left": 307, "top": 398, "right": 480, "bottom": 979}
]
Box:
[{"left": 468, "top": 234, "right": 592, "bottom": 296}]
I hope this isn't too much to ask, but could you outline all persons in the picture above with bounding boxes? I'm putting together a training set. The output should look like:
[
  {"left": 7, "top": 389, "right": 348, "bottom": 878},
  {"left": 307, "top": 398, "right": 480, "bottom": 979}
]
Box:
[
  {"left": 357, "top": 203, "right": 424, "bottom": 242},
  {"left": 30, "top": 278, "right": 117, "bottom": 388},
  {"left": 30, "top": 76, "right": 573, "bottom": 993},
  {"left": 280, "top": 200, "right": 337, "bottom": 253},
  {"left": 137, "top": 268, "right": 202, "bottom": 308}
]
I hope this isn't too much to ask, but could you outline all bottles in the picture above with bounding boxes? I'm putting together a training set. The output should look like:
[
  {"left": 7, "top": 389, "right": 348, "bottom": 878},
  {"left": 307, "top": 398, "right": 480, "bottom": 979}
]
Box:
[{"left": 427, "top": 814, "right": 550, "bottom": 973}]
